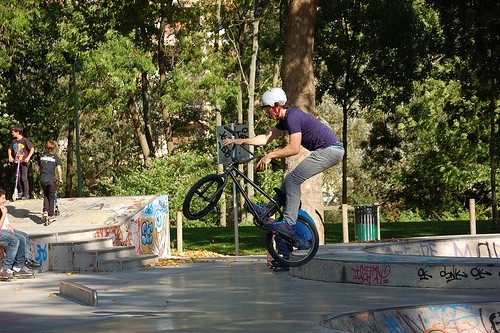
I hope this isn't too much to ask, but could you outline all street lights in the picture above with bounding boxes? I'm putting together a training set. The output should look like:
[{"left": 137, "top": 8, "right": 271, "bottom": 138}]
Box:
[{"left": 61, "top": 50, "right": 83, "bottom": 197}]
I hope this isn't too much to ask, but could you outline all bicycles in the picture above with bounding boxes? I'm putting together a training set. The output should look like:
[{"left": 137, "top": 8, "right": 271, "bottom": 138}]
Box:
[{"left": 182, "top": 124, "right": 320, "bottom": 267}]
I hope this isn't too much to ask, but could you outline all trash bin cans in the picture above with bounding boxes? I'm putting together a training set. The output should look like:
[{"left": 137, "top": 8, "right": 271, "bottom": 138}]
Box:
[{"left": 351, "top": 205, "right": 382, "bottom": 242}]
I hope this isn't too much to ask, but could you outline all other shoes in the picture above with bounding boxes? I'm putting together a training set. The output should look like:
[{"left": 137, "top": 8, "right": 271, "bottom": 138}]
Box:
[
  {"left": 25, "top": 258, "right": 40, "bottom": 270},
  {"left": 265, "top": 219, "right": 295, "bottom": 239},
  {"left": 13, "top": 267, "right": 34, "bottom": 277},
  {"left": 41, "top": 212, "right": 48, "bottom": 222},
  {"left": 244, "top": 201, "right": 268, "bottom": 226},
  {"left": 49, "top": 215, "right": 57, "bottom": 222},
  {"left": 0, "top": 269, "right": 13, "bottom": 280}
]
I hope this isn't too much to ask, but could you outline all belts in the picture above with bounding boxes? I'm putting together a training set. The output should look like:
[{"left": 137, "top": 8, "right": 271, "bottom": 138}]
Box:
[{"left": 331, "top": 143, "right": 342, "bottom": 146}]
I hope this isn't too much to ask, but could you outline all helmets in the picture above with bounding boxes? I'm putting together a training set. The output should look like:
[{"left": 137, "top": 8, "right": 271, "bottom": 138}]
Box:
[{"left": 261, "top": 88, "right": 288, "bottom": 107}]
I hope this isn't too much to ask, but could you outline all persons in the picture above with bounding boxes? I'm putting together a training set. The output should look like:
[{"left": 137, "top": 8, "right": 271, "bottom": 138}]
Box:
[
  {"left": 8, "top": 126, "right": 34, "bottom": 199},
  {"left": 37, "top": 141, "right": 62, "bottom": 221},
  {"left": 272, "top": 200, "right": 316, "bottom": 270},
  {"left": 223, "top": 88, "right": 345, "bottom": 238},
  {"left": 0, "top": 189, "right": 34, "bottom": 278}
]
